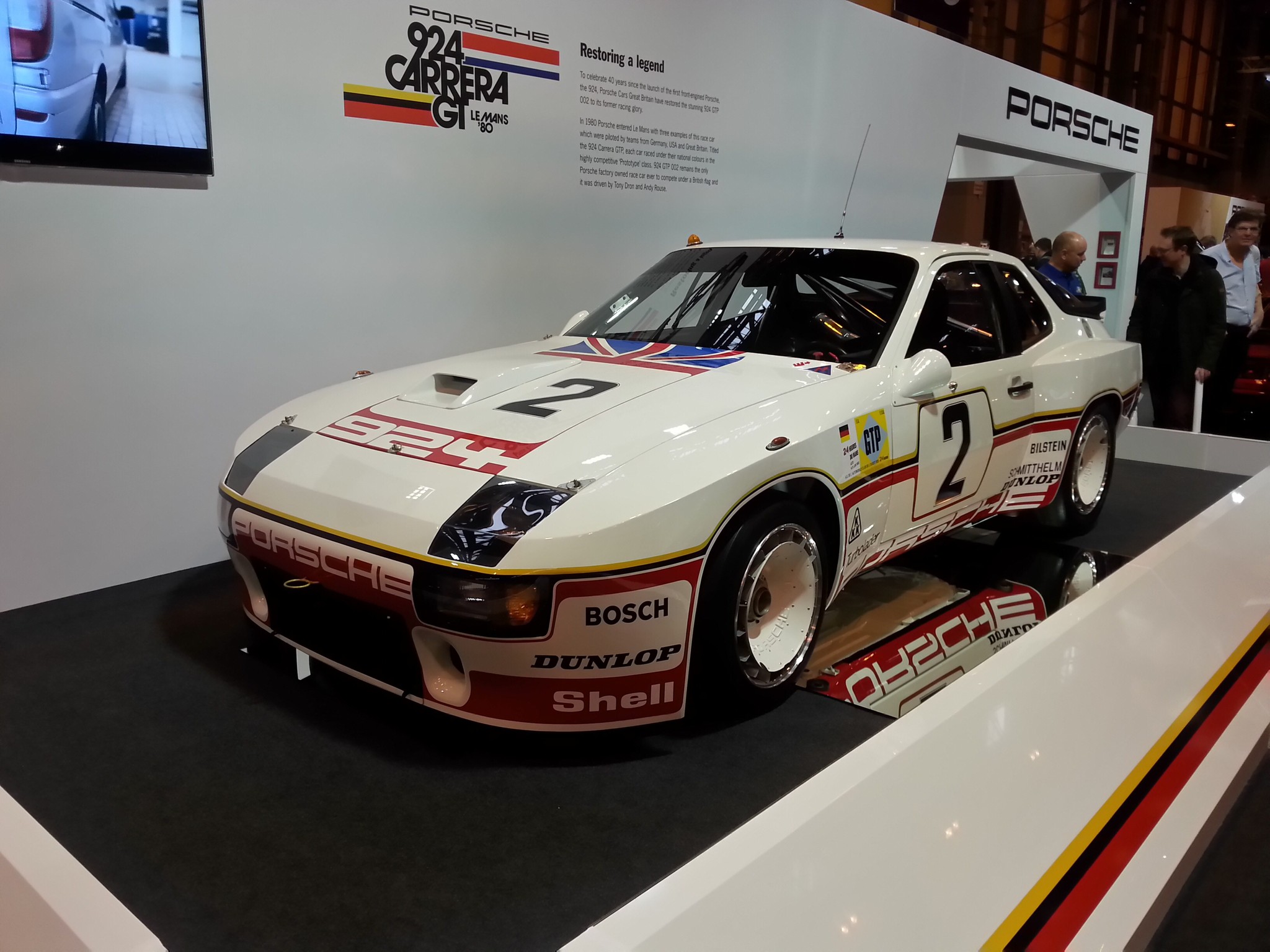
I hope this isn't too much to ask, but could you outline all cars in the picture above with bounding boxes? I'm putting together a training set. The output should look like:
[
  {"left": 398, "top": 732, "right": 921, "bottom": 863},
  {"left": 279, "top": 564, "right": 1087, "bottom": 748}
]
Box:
[{"left": 217, "top": 233, "right": 1146, "bottom": 737}]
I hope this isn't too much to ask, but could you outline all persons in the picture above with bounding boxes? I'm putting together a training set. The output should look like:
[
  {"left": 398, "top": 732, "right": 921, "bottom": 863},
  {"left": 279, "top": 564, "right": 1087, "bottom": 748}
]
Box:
[{"left": 951, "top": 202, "right": 1269, "bottom": 433}]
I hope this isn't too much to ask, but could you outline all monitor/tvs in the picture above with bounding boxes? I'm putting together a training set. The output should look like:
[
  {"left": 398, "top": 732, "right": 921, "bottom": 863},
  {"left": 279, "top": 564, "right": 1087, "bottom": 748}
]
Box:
[{"left": 1, "top": 0, "right": 217, "bottom": 179}]
